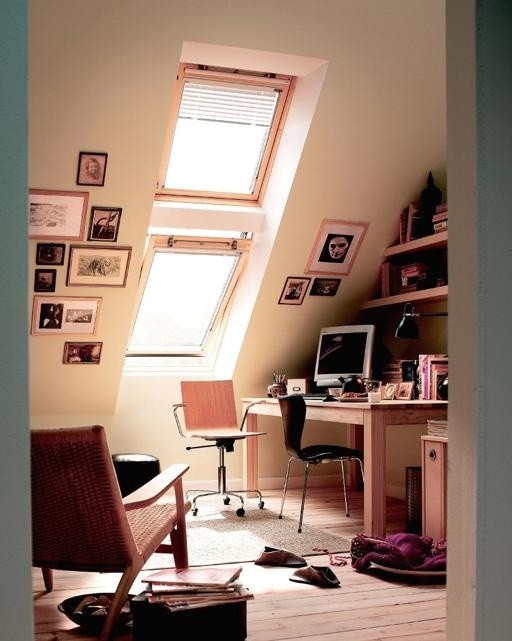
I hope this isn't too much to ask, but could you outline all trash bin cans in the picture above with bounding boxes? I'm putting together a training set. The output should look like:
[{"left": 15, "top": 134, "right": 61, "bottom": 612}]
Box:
[{"left": 405, "top": 467, "right": 422, "bottom": 533}]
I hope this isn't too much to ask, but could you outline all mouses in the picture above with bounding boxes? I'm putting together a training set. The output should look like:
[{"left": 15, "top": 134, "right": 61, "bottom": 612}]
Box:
[{"left": 323, "top": 397, "right": 339, "bottom": 402}]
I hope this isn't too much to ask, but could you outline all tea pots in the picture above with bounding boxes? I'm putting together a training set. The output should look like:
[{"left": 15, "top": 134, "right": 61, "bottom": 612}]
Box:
[{"left": 338, "top": 376, "right": 370, "bottom": 394}]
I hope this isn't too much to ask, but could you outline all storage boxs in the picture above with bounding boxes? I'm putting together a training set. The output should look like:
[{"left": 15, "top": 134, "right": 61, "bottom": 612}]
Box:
[{"left": 128, "top": 593, "right": 246, "bottom": 640}]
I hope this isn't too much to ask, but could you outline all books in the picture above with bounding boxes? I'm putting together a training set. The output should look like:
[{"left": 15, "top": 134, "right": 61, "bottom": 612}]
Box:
[
  {"left": 383, "top": 353, "right": 448, "bottom": 400},
  {"left": 304, "top": 398, "right": 323, "bottom": 401},
  {"left": 381, "top": 261, "right": 395, "bottom": 297},
  {"left": 302, "top": 393, "right": 327, "bottom": 400},
  {"left": 140, "top": 565, "right": 254, "bottom": 613},
  {"left": 400, "top": 199, "right": 448, "bottom": 244}
]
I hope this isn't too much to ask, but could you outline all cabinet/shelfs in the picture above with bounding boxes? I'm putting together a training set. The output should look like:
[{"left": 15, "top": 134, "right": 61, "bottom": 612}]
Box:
[
  {"left": 419, "top": 436, "right": 449, "bottom": 544},
  {"left": 358, "top": 231, "right": 448, "bottom": 310}
]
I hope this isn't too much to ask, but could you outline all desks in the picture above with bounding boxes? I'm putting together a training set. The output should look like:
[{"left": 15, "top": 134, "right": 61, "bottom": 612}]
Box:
[{"left": 241, "top": 397, "right": 442, "bottom": 538}]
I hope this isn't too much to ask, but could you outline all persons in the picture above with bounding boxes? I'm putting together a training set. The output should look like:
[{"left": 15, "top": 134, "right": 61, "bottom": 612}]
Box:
[
  {"left": 83, "top": 156, "right": 101, "bottom": 180},
  {"left": 327, "top": 235, "right": 350, "bottom": 260}
]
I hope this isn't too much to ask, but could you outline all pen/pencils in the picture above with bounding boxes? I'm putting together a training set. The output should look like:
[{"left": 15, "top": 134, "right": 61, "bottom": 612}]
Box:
[{"left": 272, "top": 371, "right": 287, "bottom": 383}]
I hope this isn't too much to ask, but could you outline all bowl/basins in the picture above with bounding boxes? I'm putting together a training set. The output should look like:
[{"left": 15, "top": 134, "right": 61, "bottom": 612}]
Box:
[{"left": 58, "top": 593, "right": 133, "bottom": 631}]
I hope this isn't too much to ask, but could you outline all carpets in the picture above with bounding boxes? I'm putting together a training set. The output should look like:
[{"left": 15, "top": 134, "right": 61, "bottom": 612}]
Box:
[{"left": 142, "top": 502, "right": 350, "bottom": 571}]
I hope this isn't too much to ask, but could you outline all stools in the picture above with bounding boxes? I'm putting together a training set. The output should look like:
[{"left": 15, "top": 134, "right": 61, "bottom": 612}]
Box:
[{"left": 111, "top": 452, "right": 161, "bottom": 502}]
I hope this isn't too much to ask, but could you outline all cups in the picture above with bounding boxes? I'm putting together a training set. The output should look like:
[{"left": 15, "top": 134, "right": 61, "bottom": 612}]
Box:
[
  {"left": 366, "top": 382, "right": 382, "bottom": 404},
  {"left": 267, "top": 383, "right": 287, "bottom": 399},
  {"left": 328, "top": 388, "right": 343, "bottom": 398}
]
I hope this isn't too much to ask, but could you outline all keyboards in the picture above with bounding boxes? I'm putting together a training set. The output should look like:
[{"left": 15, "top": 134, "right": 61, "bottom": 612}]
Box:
[{"left": 277, "top": 393, "right": 328, "bottom": 400}]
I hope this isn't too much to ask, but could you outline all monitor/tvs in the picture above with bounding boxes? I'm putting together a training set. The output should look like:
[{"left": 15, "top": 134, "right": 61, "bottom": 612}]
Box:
[{"left": 313, "top": 324, "right": 394, "bottom": 387}]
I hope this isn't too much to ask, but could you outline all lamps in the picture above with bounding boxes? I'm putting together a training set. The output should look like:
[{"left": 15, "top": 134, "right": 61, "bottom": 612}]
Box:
[{"left": 393, "top": 303, "right": 446, "bottom": 340}]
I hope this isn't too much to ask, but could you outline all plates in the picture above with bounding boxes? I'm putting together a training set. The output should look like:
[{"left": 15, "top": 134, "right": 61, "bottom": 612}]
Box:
[{"left": 334, "top": 397, "right": 367, "bottom": 402}]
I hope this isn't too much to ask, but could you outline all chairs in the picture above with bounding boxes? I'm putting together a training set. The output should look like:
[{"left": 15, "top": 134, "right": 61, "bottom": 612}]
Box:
[
  {"left": 172, "top": 378, "right": 269, "bottom": 517},
  {"left": 273, "top": 394, "right": 363, "bottom": 533},
  {"left": 31, "top": 425, "right": 191, "bottom": 641}
]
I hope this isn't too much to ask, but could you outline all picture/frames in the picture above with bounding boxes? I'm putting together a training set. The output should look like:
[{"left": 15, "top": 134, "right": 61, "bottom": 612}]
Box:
[
  {"left": 278, "top": 277, "right": 312, "bottom": 305},
  {"left": 304, "top": 218, "right": 371, "bottom": 277},
  {"left": 309, "top": 278, "right": 342, "bottom": 296},
  {"left": 27, "top": 150, "right": 133, "bottom": 363},
  {"left": 398, "top": 381, "right": 414, "bottom": 400}
]
